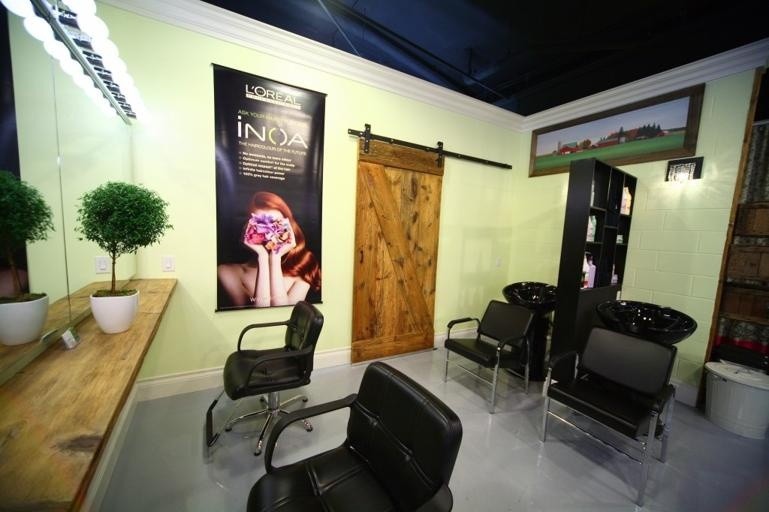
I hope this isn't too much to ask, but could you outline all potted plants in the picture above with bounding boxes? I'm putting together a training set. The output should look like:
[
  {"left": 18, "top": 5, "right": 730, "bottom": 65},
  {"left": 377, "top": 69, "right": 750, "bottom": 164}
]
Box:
[
  {"left": 0, "top": 169, "right": 56, "bottom": 347},
  {"left": 71, "top": 178, "right": 175, "bottom": 335}
]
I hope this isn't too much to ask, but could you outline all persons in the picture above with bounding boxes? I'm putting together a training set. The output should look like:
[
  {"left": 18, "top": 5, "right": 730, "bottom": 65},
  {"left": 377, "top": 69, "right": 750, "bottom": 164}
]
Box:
[{"left": 218, "top": 188, "right": 322, "bottom": 309}]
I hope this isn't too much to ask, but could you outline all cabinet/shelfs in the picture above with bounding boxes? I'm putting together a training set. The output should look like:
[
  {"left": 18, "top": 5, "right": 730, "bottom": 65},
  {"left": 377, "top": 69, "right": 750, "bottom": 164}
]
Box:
[
  {"left": 548, "top": 157, "right": 638, "bottom": 367},
  {"left": 693, "top": 66, "right": 768, "bottom": 414}
]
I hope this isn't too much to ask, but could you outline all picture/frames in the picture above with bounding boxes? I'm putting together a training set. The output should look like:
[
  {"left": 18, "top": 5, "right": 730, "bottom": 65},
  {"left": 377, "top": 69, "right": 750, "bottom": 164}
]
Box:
[{"left": 528, "top": 82, "right": 705, "bottom": 178}]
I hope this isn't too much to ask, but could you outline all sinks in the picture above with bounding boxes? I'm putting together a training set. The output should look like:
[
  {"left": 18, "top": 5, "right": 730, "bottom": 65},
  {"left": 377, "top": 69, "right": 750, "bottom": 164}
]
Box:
[
  {"left": 502, "top": 280, "right": 558, "bottom": 313},
  {"left": 594, "top": 298, "right": 697, "bottom": 345}
]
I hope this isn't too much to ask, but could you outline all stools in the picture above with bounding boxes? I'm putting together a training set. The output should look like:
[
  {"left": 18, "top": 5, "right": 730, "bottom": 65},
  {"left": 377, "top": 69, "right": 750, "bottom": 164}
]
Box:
[{"left": 502, "top": 281, "right": 697, "bottom": 439}]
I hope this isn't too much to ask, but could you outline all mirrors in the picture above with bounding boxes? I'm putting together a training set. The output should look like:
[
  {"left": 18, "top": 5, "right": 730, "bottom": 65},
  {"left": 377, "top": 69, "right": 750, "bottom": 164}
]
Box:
[{"left": 0, "top": 0, "right": 138, "bottom": 385}]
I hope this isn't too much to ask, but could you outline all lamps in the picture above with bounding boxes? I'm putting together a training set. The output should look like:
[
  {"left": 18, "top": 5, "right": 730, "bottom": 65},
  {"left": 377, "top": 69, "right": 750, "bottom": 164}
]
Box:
[
  {"left": 1, "top": 0, "right": 117, "bottom": 120},
  {"left": 46, "top": 0, "right": 147, "bottom": 120}
]
